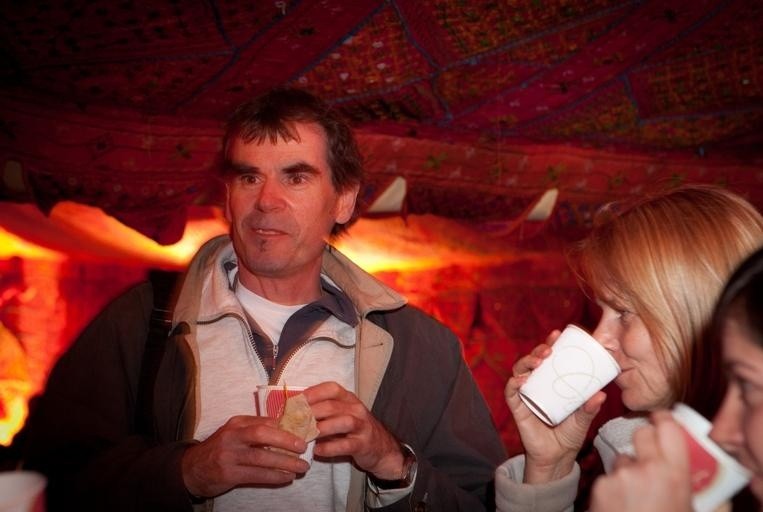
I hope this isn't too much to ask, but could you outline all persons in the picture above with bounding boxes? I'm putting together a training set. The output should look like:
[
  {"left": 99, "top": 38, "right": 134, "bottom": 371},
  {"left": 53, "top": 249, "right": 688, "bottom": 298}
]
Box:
[
  {"left": 493, "top": 184, "right": 763, "bottom": 512},
  {"left": 591, "top": 245, "right": 763, "bottom": 511},
  {"left": 16, "top": 90, "right": 507, "bottom": 511}
]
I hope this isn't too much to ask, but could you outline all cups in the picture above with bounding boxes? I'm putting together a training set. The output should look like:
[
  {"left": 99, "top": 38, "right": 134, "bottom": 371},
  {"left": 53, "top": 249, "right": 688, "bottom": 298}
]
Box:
[
  {"left": 256, "top": 382, "right": 316, "bottom": 473},
  {"left": 0, "top": 469, "right": 48, "bottom": 510},
  {"left": 517, "top": 323, "right": 622, "bottom": 429},
  {"left": 672, "top": 400, "right": 754, "bottom": 512}
]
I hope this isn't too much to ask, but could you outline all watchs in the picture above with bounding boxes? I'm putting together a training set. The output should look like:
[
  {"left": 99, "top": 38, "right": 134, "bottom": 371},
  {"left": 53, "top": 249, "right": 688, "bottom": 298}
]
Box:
[{"left": 378, "top": 448, "right": 418, "bottom": 489}]
